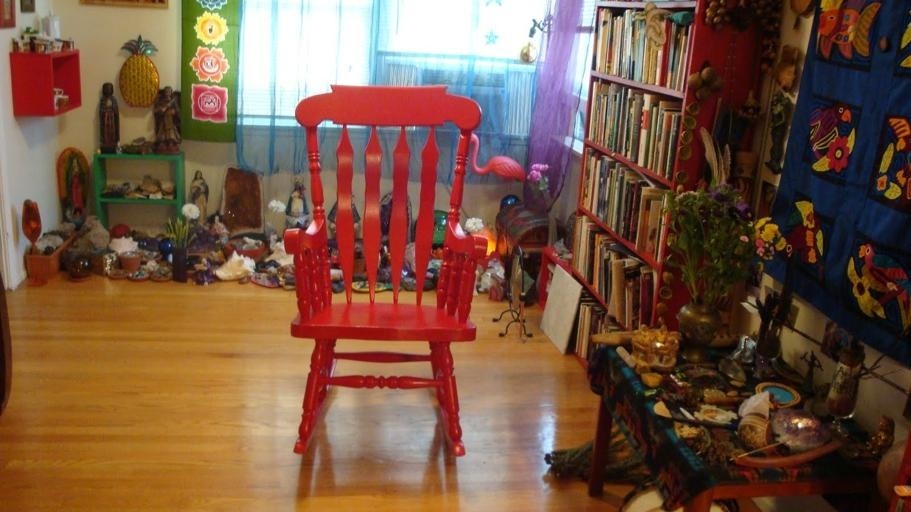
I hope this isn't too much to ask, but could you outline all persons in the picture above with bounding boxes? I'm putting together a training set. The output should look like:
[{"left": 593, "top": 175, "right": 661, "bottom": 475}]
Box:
[
  {"left": 153, "top": 85, "right": 181, "bottom": 146},
  {"left": 97, "top": 81, "right": 123, "bottom": 153},
  {"left": 191, "top": 169, "right": 210, "bottom": 224},
  {"left": 65, "top": 153, "right": 88, "bottom": 219}
]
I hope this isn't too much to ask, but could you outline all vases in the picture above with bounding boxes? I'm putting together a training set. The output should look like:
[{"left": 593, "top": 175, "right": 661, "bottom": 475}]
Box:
[
  {"left": 672, "top": 295, "right": 726, "bottom": 364},
  {"left": 170, "top": 245, "right": 189, "bottom": 285}
]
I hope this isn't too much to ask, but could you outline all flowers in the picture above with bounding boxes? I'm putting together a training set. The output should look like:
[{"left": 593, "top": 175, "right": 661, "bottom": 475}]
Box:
[
  {"left": 186, "top": 44, "right": 233, "bottom": 84},
  {"left": 193, "top": 9, "right": 232, "bottom": 47},
  {"left": 524, "top": 160, "right": 560, "bottom": 245},
  {"left": 167, "top": 199, "right": 201, "bottom": 249},
  {"left": 652, "top": 177, "right": 791, "bottom": 317},
  {"left": 822, "top": 133, "right": 854, "bottom": 175},
  {"left": 264, "top": 196, "right": 287, "bottom": 235},
  {"left": 464, "top": 215, "right": 487, "bottom": 237}
]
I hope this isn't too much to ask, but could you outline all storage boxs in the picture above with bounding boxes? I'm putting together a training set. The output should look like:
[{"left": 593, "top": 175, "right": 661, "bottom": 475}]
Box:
[{"left": 494, "top": 201, "right": 569, "bottom": 309}]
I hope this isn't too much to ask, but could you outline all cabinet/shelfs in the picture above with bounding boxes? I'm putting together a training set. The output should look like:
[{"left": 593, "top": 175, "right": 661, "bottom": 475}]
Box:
[
  {"left": 9, "top": 45, "right": 85, "bottom": 121},
  {"left": 564, "top": 1, "right": 770, "bottom": 391},
  {"left": 91, "top": 147, "right": 189, "bottom": 239}
]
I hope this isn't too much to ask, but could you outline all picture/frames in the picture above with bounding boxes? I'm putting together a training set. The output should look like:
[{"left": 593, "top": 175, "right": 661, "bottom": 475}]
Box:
[
  {"left": 18, "top": 0, "right": 38, "bottom": 14},
  {"left": 78, "top": 1, "right": 171, "bottom": 13},
  {"left": 0, "top": 0, "right": 16, "bottom": 31}
]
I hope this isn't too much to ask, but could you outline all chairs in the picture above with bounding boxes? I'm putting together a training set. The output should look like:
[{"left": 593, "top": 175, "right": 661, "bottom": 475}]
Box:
[{"left": 277, "top": 79, "right": 492, "bottom": 462}]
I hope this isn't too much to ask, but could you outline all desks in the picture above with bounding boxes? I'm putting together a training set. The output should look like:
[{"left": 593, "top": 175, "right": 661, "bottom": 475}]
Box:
[{"left": 585, "top": 322, "right": 879, "bottom": 511}]
